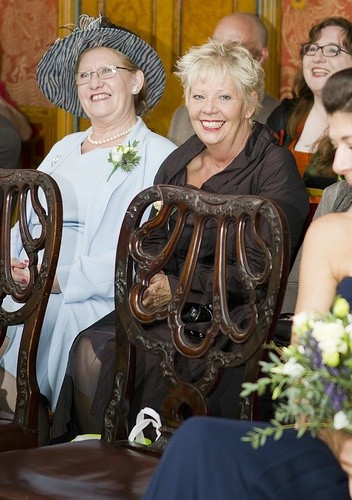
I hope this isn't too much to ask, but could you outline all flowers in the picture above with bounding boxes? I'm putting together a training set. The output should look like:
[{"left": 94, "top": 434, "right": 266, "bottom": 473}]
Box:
[
  {"left": 105, "top": 139, "right": 140, "bottom": 182},
  {"left": 241, "top": 296, "right": 352, "bottom": 450}
]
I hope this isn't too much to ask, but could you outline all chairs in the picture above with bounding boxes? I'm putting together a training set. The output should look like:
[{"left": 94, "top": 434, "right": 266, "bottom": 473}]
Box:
[
  {"left": 0, "top": 168, "right": 63, "bottom": 452},
  {"left": 0, "top": 185, "right": 289, "bottom": 500}
]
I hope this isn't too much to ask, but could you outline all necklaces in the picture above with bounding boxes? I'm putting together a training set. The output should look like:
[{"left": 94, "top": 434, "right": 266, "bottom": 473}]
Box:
[{"left": 88, "top": 127, "right": 132, "bottom": 145}]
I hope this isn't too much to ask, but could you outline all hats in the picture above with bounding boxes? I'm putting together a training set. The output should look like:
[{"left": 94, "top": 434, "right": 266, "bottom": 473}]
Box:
[{"left": 34, "top": 13, "right": 167, "bottom": 115}]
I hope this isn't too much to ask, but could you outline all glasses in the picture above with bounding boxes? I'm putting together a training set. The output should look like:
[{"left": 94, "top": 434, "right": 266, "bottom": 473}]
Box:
[
  {"left": 301, "top": 43, "right": 352, "bottom": 58},
  {"left": 73, "top": 65, "right": 136, "bottom": 85}
]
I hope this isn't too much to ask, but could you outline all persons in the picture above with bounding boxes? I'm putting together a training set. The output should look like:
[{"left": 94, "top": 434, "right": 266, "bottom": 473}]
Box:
[
  {"left": 165, "top": 11, "right": 279, "bottom": 150},
  {"left": 270, "top": 17, "right": 352, "bottom": 230},
  {"left": 0, "top": 12, "right": 180, "bottom": 419},
  {"left": 279, "top": 131, "right": 352, "bottom": 318},
  {"left": 158, "top": 67, "right": 352, "bottom": 500},
  {"left": 49, "top": 43, "right": 309, "bottom": 445}
]
